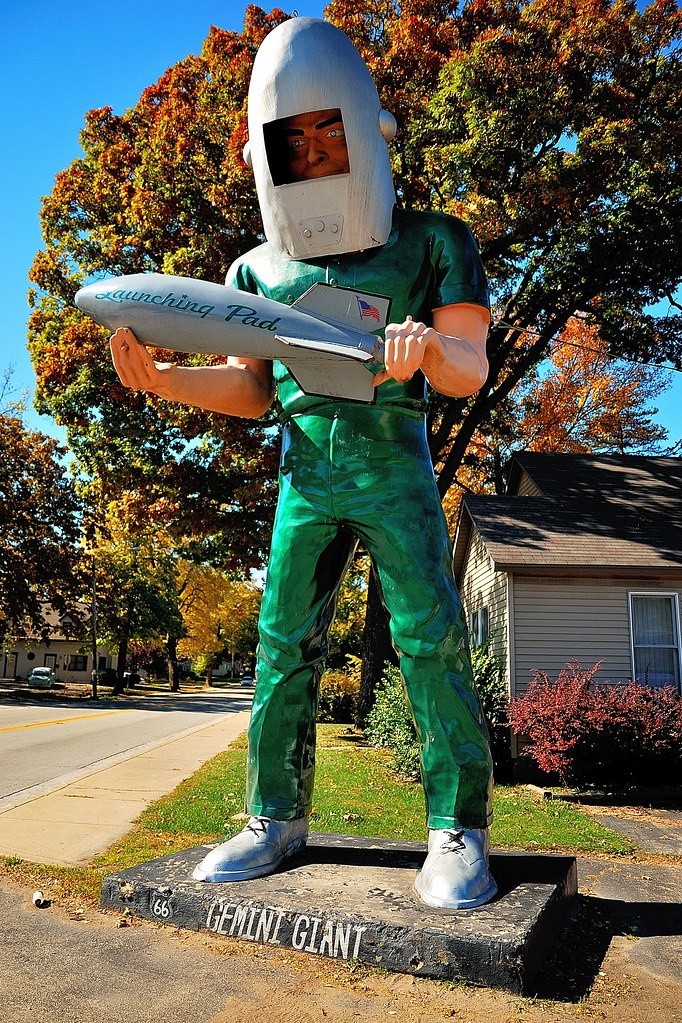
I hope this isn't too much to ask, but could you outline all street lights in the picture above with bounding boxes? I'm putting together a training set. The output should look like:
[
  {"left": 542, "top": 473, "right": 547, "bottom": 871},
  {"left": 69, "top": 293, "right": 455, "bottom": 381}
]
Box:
[{"left": 92, "top": 546, "right": 140, "bottom": 698}]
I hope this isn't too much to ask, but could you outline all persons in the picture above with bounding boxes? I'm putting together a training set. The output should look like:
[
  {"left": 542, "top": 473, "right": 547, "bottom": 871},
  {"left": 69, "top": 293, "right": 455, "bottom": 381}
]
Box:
[{"left": 113, "top": 18, "right": 498, "bottom": 906}]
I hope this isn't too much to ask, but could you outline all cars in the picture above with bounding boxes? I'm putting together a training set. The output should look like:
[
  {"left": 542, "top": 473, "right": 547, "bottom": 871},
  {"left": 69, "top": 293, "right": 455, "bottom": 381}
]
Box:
[
  {"left": 241, "top": 676, "right": 257, "bottom": 687},
  {"left": 28, "top": 666, "right": 57, "bottom": 688}
]
